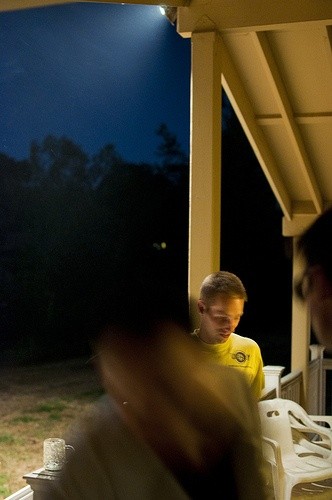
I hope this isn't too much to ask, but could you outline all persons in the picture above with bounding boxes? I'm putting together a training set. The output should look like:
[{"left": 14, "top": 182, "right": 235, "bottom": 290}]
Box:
[
  {"left": 52, "top": 271, "right": 265, "bottom": 500},
  {"left": 137, "top": 205, "right": 332, "bottom": 500},
  {"left": 186, "top": 271, "right": 266, "bottom": 402}
]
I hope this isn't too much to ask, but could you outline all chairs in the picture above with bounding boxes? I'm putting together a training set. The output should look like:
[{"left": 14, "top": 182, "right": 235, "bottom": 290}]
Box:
[{"left": 256, "top": 398, "right": 332, "bottom": 500}]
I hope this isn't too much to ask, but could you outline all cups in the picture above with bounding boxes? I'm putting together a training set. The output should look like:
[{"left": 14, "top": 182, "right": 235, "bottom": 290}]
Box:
[{"left": 43, "top": 437, "right": 76, "bottom": 472}]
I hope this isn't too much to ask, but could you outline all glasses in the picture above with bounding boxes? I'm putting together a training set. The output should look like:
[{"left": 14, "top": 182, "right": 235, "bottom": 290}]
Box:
[{"left": 292, "top": 268, "right": 324, "bottom": 300}]
